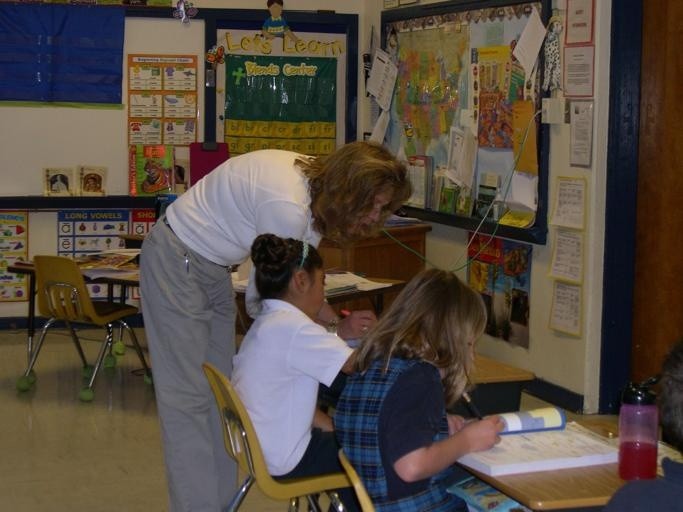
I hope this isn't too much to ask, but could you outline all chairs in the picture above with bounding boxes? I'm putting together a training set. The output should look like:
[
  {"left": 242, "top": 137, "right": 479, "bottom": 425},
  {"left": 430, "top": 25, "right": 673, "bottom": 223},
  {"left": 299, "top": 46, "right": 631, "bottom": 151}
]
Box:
[
  {"left": 201, "top": 363, "right": 376, "bottom": 512},
  {"left": 20, "top": 253, "right": 152, "bottom": 401}
]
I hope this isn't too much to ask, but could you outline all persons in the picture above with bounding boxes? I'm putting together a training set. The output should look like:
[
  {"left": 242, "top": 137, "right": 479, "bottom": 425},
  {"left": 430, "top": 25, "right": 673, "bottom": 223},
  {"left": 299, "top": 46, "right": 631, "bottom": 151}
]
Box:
[
  {"left": 138, "top": 140, "right": 415, "bottom": 512},
  {"left": 332, "top": 266, "right": 507, "bottom": 512},
  {"left": 226, "top": 231, "right": 362, "bottom": 511},
  {"left": 600, "top": 337, "right": 683, "bottom": 511}
]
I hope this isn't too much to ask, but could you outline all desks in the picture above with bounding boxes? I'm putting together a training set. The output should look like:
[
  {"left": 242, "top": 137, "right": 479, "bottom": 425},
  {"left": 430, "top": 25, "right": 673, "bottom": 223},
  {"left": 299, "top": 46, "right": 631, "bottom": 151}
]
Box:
[
  {"left": 7, "top": 268, "right": 406, "bottom": 373},
  {"left": 445, "top": 350, "right": 683, "bottom": 512}
]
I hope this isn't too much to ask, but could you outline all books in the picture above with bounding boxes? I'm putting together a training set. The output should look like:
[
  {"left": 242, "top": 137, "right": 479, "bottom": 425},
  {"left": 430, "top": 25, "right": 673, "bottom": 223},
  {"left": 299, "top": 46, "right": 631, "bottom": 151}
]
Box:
[
  {"left": 42, "top": 165, "right": 75, "bottom": 198},
  {"left": 74, "top": 164, "right": 109, "bottom": 198},
  {"left": 433, "top": 405, "right": 620, "bottom": 477},
  {"left": 14, "top": 246, "right": 141, "bottom": 280},
  {"left": 497, "top": 209, "right": 535, "bottom": 229},
  {"left": 445, "top": 476, "right": 519, "bottom": 512},
  {"left": 407, "top": 153, "right": 474, "bottom": 218}
]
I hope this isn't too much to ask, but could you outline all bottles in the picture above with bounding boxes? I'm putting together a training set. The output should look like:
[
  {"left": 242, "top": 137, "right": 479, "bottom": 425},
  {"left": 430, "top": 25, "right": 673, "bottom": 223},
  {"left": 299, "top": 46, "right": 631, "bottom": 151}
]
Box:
[{"left": 617, "top": 373, "right": 663, "bottom": 482}]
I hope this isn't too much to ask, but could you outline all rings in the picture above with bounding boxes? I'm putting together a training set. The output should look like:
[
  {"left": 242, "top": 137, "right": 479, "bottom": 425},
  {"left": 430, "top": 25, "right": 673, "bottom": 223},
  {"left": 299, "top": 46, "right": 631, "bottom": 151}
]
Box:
[{"left": 363, "top": 326, "right": 370, "bottom": 333}]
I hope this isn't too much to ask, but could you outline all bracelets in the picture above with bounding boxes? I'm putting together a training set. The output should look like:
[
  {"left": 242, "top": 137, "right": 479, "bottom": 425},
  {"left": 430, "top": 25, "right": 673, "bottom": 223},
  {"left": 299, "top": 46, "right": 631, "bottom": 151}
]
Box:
[{"left": 329, "top": 319, "right": 338, "bottom": 334}]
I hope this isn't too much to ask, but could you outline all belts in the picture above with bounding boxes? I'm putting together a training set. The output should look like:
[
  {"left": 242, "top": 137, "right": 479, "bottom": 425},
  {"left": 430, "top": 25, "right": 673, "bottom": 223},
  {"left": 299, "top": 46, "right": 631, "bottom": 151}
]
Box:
[{"left": 163, "top": 216, "right": 240, "bottom": 273}]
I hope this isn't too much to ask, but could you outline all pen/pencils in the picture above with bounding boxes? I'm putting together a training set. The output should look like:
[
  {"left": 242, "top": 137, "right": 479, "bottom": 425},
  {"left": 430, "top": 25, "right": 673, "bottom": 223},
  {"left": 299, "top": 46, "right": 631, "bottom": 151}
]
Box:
[
  {"left": 462, "top": 392, "right": 483, "bottom": 421},
  {"left": 340, "top": 309, "right": 352, "bottom": 317}
]
